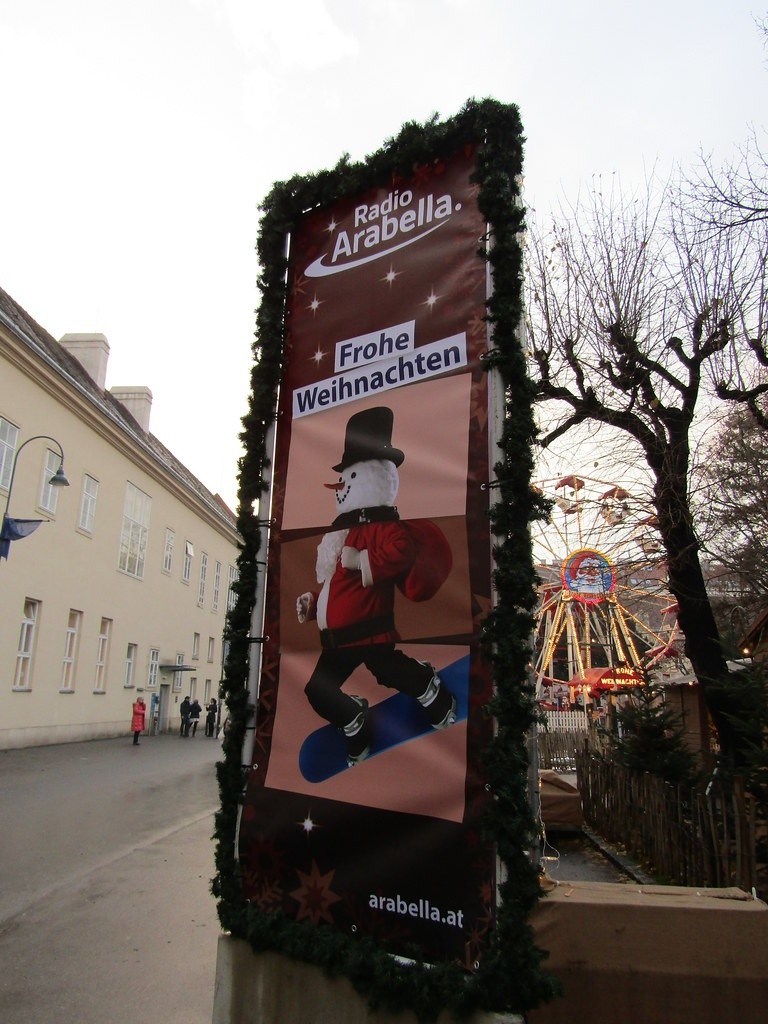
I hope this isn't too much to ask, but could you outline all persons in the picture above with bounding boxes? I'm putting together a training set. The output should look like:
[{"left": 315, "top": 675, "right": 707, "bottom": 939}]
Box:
[
  {"left": 131, "top": 697, "right": 146, "bottom": 745},
  {"left": 206, "top": 698, "right": 217, "bottom": 737},
  {"left": 189, "top": 700, "right": 202, "bottom": 738},
  {"left": 180, "top": 696, "right": 191, "bottom": 737}
]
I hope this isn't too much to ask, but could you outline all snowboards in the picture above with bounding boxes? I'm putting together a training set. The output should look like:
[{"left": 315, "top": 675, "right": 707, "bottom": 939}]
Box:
[{"left": 299, "top": 653, "right": 473, "bottom": 785}]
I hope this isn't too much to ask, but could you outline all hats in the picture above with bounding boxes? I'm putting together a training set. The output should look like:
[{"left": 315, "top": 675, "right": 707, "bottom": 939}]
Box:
[{"left": 185, "top": 696, "right": 190, "bottom": 699}]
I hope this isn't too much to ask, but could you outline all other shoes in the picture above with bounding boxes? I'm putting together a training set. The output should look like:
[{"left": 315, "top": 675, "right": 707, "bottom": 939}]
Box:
[{"left": 134, "top": 743, "right": 140, "bottom": 745}]
